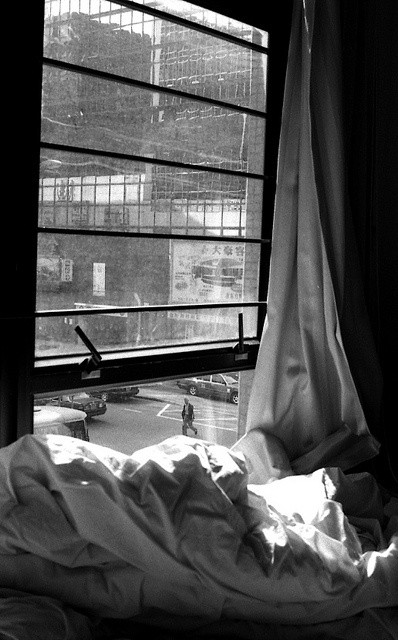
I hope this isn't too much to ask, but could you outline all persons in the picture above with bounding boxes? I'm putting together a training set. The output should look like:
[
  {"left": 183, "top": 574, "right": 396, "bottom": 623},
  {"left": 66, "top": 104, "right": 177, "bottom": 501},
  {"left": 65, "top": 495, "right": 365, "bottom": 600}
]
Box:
[{"left": 181, "top": 398, "right": 198, "bottom": 436}]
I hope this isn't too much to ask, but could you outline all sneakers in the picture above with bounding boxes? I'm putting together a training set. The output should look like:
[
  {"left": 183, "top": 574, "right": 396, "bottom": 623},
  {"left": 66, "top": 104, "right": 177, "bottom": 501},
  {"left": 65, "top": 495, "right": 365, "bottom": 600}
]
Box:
[{"left": 195, "top": 429, "right": 197, "bottom": 435}]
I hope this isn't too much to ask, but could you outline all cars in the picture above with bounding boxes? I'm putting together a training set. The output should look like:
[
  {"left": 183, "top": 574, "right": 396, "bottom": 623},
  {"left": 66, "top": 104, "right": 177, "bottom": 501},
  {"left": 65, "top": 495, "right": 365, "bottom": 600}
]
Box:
[
  {"left": 89, "top": 387, "right": 139, "bottom": 402},
  {"left": 176, "top": 372, "right": 239, "bottom": 405},
  {"left": 45, "top": 391, "right": 107, "bottom": 420}
]
